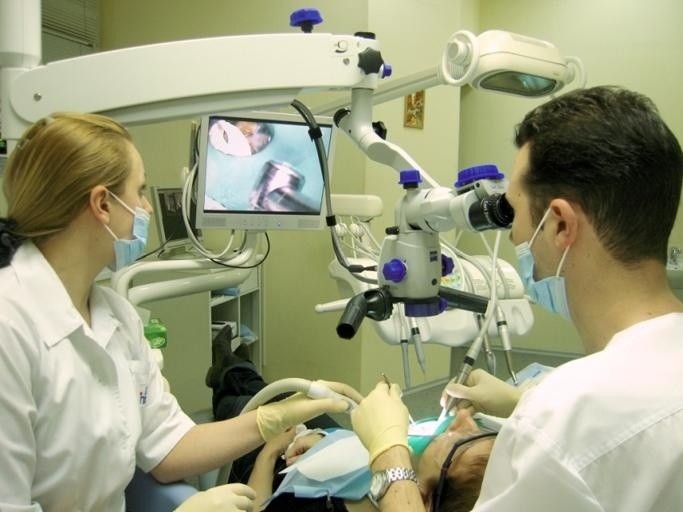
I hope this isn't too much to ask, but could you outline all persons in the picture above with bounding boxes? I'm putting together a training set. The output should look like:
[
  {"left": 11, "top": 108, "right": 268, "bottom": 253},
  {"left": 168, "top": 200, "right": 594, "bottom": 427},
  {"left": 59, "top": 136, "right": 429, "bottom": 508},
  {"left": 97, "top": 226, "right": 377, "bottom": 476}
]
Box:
[
  {"left": 1, "top": 109, "right": 358, "bottom": 509},
  {"left": 204, "top": 324, "right": 496, "bottom": 510},
  {"left": 349, "top": 87, "right": 681, "bottom": 509}
]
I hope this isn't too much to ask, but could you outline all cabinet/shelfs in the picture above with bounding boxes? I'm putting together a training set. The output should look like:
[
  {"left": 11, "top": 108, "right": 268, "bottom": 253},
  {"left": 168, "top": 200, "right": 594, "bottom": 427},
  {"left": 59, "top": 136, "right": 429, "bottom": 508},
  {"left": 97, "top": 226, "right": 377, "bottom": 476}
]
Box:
[{"left": 213, "top": 264, "right": 260, "bottom": 383}]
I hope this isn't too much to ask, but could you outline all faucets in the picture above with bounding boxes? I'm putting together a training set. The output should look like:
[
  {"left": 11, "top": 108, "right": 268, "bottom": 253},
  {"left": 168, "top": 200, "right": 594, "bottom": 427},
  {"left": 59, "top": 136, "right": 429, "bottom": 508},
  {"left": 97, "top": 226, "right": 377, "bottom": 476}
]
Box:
[{"left": 669, "top": 246, "right": 680, "bottom": 264}]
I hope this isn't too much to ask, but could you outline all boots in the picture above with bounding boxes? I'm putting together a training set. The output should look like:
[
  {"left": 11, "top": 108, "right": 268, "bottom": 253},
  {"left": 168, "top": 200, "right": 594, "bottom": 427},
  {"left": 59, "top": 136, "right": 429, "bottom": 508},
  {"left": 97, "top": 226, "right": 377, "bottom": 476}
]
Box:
[{"left": 206, "top": 324, "right": 258, "bottom": 415}]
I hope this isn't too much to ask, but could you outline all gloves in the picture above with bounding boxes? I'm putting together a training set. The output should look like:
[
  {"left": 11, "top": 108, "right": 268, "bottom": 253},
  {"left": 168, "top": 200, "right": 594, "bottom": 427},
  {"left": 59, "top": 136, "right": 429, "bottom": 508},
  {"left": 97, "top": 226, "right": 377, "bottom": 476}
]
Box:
[
  {"left": 440, "top": 368, "right": 523, "bottom": 418},
  {"left": 171, "top": 483, "right": 257, "bottom": 512},
  {"left": 257, "top": 380, "right": 364, "bottom": 443},
  {"left": 352, "top": 382, "right": 411, "bottom": 469}
]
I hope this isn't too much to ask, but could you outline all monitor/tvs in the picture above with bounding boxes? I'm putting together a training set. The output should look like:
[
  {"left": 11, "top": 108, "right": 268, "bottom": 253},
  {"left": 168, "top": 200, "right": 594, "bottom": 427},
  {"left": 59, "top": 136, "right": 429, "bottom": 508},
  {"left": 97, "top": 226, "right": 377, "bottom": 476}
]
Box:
[{"left": 196, "top": 110, "right": 334, "bottom": 229}]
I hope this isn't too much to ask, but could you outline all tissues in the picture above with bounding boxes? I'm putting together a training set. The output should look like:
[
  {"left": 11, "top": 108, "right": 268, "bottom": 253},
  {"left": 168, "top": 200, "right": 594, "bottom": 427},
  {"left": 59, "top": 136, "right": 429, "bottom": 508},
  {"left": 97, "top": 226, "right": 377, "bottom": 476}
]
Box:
[{"left": 134, "top": 307, "right": 167, "bottom": 349}]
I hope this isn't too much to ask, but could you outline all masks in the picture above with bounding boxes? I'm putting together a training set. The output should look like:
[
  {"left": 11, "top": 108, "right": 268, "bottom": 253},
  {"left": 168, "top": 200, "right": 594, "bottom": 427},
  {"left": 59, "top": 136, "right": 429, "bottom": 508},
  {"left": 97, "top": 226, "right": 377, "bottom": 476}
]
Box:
[
  {"left": 515, "top": 206, "right": 572, "bottom": 322},
  {"left": 98, "top": 192, "right": 150, "bottom": 273}
]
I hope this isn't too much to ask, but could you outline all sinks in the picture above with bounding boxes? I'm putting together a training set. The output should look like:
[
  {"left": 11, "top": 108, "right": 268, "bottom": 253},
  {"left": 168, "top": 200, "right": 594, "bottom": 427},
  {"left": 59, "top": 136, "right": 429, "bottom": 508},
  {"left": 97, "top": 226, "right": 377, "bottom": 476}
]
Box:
[{"left": 666, "top": 266, "right": 683, "bottom": 289}]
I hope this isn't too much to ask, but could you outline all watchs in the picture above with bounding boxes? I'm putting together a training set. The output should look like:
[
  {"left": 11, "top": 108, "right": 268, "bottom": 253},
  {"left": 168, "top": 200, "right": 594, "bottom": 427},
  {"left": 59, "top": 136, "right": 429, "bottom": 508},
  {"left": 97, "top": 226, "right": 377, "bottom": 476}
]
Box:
[{"left": 363, "top": 462, "right": 419, "bottom": 503}]
